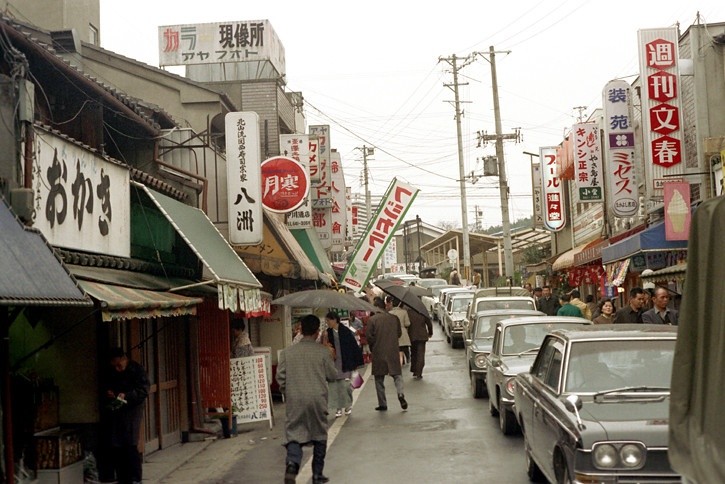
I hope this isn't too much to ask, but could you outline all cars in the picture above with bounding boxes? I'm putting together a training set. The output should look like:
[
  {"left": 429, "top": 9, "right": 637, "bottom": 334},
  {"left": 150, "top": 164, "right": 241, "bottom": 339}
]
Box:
[{"left": 513, "top": 323, "right": 682, "bottom": 484}]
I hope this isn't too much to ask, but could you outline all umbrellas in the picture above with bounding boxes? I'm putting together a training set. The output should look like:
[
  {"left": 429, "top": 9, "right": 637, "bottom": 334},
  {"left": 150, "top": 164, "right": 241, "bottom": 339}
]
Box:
[
  {"left": 421, "top": 267, "right": 436, "bottom": 273},
  {"left": 406, "top": 285, "right": 435, "bottom": 296},
  {"left": 372, "top": 278, "right": 404, "bottom": 284},
  {"left": 375, "top": 282, "right": 431, "bottom": 319},
  {"left": 270, "top": 290, "right": 381, "bottom": 313}
]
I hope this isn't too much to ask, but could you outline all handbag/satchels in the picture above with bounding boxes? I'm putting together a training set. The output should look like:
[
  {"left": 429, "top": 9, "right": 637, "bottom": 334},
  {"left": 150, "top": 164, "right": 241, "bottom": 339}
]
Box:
[
  {"left": 350, "top": 373, "right": 364, "bottom": 389},
  {"left": 322, "top": 333, "right": 336, "bottom": 361}
]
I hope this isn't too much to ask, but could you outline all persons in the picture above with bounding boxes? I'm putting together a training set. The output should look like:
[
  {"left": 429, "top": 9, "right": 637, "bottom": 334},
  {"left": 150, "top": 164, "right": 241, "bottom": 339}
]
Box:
[
  {"left": 525, "top": 282, "right": 679, "bottom": 326},
  {"left": 100, "top": 348, "right": 150, "bottom": 484},
  {"left": 504, "top": 325, "right": 537, "bottom": 353},
  {"left": 567, "top": 351, "right": 599, "bottom": 392},
  {"left": 276, "top": 314, "right": 338, "bottom": 484},
  {"left": 231, "top": 318, "right": 253, "bottom": 358},
  {"left": 482, "top": 318, "right": 500, "bottom": 337},
  {"left": 9, "top": 361, "right": 40, "bottom": 478},
  {"left": 429, "top": 268, "right": 459, "bottom": 284},
  {"left": 292, "top": 296, "right": 433, "bottom": 416},
  {"left": 455, "top": 300, "right": 468, "bottom": 311}
]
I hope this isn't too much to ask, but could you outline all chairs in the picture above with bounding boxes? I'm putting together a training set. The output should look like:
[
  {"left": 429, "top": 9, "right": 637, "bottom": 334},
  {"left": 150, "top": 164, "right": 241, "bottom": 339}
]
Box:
[{"left": 582, "top": 362, "right": 617, "bottom": 392}]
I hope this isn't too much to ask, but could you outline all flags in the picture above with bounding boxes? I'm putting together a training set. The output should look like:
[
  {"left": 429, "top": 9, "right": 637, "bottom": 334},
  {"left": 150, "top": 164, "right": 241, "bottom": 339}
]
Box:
[{"left": 339, "top": 179, "right": 420, "bottom": 294}]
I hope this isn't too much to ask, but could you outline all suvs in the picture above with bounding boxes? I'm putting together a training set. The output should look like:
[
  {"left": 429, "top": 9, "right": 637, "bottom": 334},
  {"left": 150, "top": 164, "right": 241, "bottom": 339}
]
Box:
[
  {"left": 414, "top": 278, "right": 478, "bottom": 350},
  {"left": 485, "top": 316, "right": 596, "bottom": 441},
  {"left": 377, "top": 273, "right": 420, "bottom": 287},
  {"left": 462, "top": 278, "right": 548, "bottom": 400}
]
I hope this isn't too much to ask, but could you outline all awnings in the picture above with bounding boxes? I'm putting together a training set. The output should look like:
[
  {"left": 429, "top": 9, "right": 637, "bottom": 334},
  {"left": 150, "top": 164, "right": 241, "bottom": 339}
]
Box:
[
  {"left": 76, "top": 280, "right": 203, "bottom": 353},
  {"left": 526, "top": 237, "right": 602, "bottom": 273},
  {"left": 232, "top": 208, "right": 338, "bottom": 280},
  {"left": 129, "top": 180, "right": 263, "bottom": 312},
  {"left": 0, "top": 194, "right": 93, "bottom": 305},
  {"left": 640, "top": 262, "right": 687, "bottom": 296},
  {"left": 574, "top": 223, "right": 645, "bottom": 267},
  {"left": 602, "top": 222, "right": 688, "bottom": 264}
]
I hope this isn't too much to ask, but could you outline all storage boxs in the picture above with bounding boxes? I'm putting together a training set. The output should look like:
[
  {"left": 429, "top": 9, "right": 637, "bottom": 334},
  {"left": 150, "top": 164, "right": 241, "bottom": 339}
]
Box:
[{"left": 34, "top": 426, "right": 86, "bottom": 470}]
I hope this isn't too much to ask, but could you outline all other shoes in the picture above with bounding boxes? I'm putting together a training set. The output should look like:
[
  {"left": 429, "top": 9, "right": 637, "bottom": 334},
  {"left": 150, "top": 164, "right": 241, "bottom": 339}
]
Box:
[
  {"left": 284, "top": 461, "right": 296, "bottom": 484},
  {"left": 413, "top": 372, "right": 417, "bottom": 376},
  {"left": 398, "top": 393, "right": 408, "bottom": 409},
  {"left": 312, "top": 474, "right": 329, "bottom": 484},
  {"left": 418, "top": 375, "right": 423, "bottom": 378},
  {"left": 375, "top": 405, "right": 387, "bottom": 411}
]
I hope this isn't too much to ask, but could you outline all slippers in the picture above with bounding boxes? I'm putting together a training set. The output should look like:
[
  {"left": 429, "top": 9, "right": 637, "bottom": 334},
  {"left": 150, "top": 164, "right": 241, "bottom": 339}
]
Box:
[
  {"left": 335, "top": 409, "right": 342, "bottom": 416},
  {"left": 345, "top": 408, "right": 351, "bottom": 415}
]
